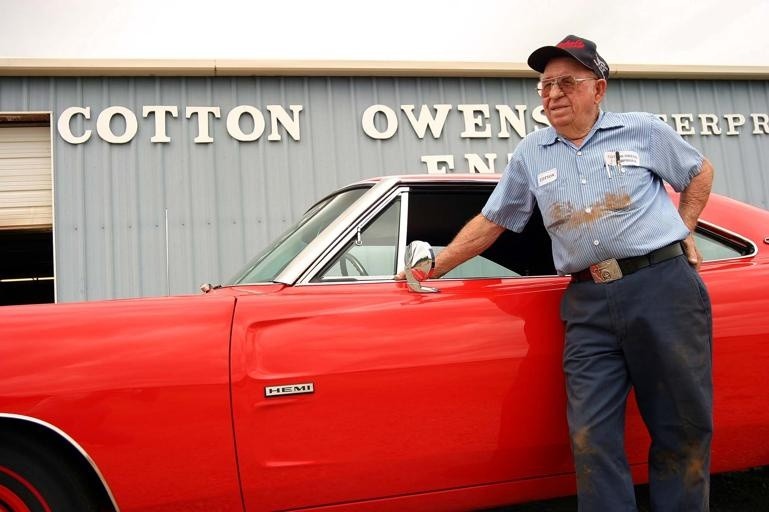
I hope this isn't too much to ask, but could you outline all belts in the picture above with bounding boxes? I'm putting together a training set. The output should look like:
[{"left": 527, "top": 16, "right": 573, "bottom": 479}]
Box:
[{"left": 570, "top": 243, "right": 685, "bottom": 286}]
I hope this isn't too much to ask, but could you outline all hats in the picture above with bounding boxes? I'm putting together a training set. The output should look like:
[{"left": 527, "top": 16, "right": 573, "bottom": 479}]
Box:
[{"left": 527, "top": 36, "right": 610, "bottom": 79}]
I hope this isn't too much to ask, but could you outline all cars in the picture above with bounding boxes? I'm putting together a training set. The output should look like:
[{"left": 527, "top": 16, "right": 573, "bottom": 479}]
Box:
[{"left": 0, "top": 175, "right": 769, "bottom": 511}]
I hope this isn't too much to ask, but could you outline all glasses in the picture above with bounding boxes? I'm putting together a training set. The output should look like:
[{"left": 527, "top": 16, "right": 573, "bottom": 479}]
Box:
[{"left": 537, "top": 74, "right": 595, "bottom": 98}]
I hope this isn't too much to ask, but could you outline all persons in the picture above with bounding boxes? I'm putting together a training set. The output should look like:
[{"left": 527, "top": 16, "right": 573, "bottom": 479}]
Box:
[{"left": 395, "top": 36, "right": 715, "bottom": 512}]
